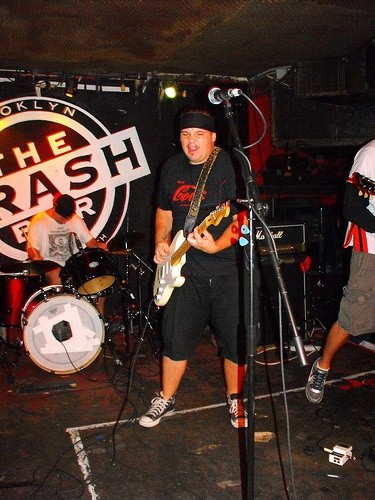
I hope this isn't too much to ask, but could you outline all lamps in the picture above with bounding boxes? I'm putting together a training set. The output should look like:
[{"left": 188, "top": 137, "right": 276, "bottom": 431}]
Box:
[{"left": 65, "top": 78, "right": 78, "bottom": 98}]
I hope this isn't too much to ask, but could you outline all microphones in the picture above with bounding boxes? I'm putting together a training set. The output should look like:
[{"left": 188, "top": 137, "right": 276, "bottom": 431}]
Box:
[{"left": 208, "top": 86, "right": 242, "bottom": 104}]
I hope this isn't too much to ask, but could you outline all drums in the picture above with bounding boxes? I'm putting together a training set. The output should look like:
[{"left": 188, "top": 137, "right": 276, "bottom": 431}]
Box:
[
  {"left": 65, "top": 247, "right": 116, "bottom": 295},
  {"left": 0, "top": 273, "right": 44, "bottom": 330},
  {"left": 20, "top": 284, "right": 105, "bottom": 375}
]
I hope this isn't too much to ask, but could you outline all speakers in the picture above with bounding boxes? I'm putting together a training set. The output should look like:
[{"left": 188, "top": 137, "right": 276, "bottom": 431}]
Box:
[{"left": 253, "top": 252, "right": 307, "bottom": 347}]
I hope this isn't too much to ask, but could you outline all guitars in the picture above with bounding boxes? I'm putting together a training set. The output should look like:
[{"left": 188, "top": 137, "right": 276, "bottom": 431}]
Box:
[{"left": 153, "top": 200, "right": 231, "bottom": 307}]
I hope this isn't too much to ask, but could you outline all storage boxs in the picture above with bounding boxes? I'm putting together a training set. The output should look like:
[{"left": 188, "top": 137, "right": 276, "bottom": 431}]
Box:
[{"left": 329, "top": 443, "right": 352, "bottom": 465}]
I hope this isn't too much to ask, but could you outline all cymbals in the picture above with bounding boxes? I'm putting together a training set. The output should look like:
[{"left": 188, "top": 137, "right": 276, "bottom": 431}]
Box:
[
  {"left": 1, "top": 261, "right": 59, "bottom": 274},
  {"left": 107, "top": 232, "right": 145, "bottom": 252}
]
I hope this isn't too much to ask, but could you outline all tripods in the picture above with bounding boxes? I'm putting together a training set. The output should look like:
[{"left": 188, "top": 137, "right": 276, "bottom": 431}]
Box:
[
  {"left": 104, "top": 241, "right": 159, "bottom": 357},
  {"left": 306, "top": 206, "right": 339, "bottom": 338}
]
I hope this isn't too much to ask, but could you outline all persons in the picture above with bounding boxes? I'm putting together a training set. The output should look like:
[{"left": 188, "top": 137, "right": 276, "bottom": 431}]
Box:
[
  {"left": 139, "top": 109, "right": 249, "bottom": 428},
  {"left": 305, "top": 138, "right": 375, "bottom": 403},
  {"left": 27, "top": 194, "right": 107, "bottom": 313}
]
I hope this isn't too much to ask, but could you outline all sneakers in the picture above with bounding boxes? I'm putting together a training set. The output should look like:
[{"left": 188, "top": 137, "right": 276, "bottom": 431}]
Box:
[
  {"left": 139, "top": 391, "right": 175, "bottom": 427},
  {"left": 228, "top": 393, "right": 248, "bottom": 428},
  {"left": 305, "top": 356, "right": 330, "bottom": 404}
]
